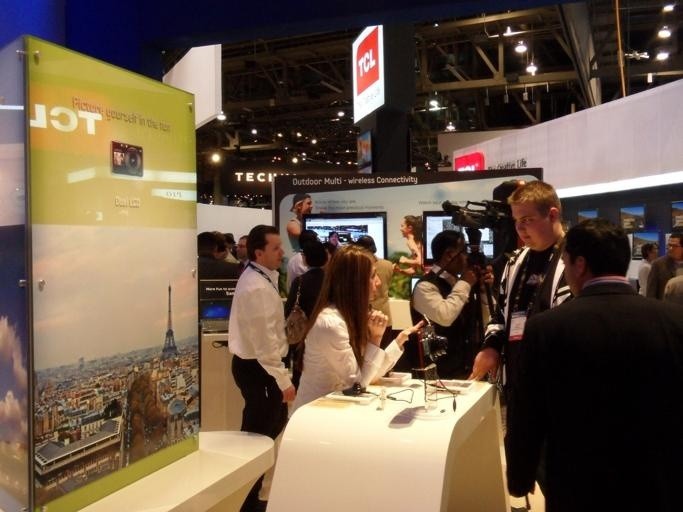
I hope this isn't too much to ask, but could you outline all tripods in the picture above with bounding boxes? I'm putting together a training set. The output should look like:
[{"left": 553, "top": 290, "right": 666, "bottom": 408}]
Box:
[{"left": 461, "top": 255, "right": 495, "bottom": 348}]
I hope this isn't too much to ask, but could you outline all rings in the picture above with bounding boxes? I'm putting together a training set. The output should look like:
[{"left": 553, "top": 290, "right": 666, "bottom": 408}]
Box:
[{"left": 379, "top": 321, "right": 383, "bottom": 324}]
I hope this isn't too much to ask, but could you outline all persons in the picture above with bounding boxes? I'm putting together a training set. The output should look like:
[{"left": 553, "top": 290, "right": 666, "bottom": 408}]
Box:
[
  {"left": 638, "top": 227, "right": 683, "bottom": 300},
  {"left": 501, "top": 216, "right": 683, "bottom": 512},
  {"left": 463, "top": 179, "right": 576, "bottom": 408},
  {"left": 410, "top": 229, "right": 495, "bottom": 378},
  {"left": 283, "top": 192, "right": 343, "bottom": 296},
  {"left": 391, "top": 215, "right": 433, "bottom": 277},
  {"left": 288, "top": 244, "right": 425, "bottom": 418},
  {"left": 357, "top": 235, "right": 394, "bottom": 349},
  {"left": 283, "top": 241, "right": 328, "bottom": 392},
  {"left": 198, "top": 230, "right": 249, "bottom": 279},
  {"left": 226, "top": 224, "right": 296, "bottom": 512}
]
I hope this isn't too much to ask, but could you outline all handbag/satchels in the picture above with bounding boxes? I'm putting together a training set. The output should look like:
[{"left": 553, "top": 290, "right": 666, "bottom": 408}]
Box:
[{"left": 284, "top": 305, "right": 309, "bottom": 344}]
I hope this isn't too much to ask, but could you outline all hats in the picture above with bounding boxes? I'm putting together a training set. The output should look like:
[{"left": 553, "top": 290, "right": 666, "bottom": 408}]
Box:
[{"left": 290, "top": 193, "right": 310, "bottom": 212}]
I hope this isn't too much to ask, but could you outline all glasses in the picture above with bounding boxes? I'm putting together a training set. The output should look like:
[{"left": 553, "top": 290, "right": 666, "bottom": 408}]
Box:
[
  {"left": 238, "top": 244, "right": 247, "bottom": 248},
  {"left": 666, "top": 243, "right": 683, "bottom": 249}
]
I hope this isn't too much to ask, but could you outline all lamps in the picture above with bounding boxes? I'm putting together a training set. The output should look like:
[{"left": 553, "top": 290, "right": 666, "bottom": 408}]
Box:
[
  {"left": 526, "top": 31, "right": 538, "bottom": 73},
  {"left": 515, "top": 40, "right": 527, "bottom": 53}
]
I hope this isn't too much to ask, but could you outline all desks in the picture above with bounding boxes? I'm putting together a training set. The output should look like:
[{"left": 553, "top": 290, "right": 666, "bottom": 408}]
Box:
[
  {"left": 200, "top": 334, "right": 246, "bottom": 430},
  {"left": 266, "top": 380, "right": 510, "bottom": 512}
]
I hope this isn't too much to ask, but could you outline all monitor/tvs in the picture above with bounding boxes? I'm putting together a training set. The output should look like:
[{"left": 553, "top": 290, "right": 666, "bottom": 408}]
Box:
[
  {"left": 577, "top": 208, "right": 600, "bottom": 224},
  {"left": 618, "top": 203, "right": 649, "bottom": 234},
  {"left": 424, "top": 209, "right": 496, "bottom": 264},
  {"left": 670, "top": 201, "right": 683, "bottom": 231},
  {"left": 631, "top": 230, "right": 661, "bottom": 260},
  {"left": 112, "top": 140, "right": 145, "bottom": 179},
  {"left": 302, "top": 210, "right": 388, "bottom": 262}
]
